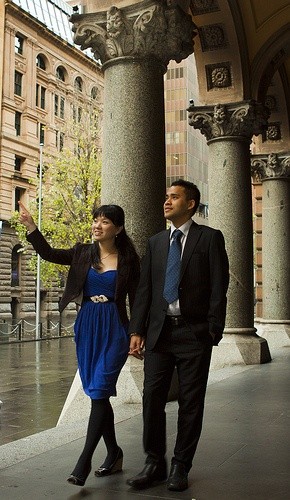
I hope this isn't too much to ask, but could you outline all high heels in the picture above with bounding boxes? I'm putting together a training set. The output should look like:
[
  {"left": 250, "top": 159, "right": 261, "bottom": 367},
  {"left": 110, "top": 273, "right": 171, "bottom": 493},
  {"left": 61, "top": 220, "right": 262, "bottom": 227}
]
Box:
[
  {"left": 67, "top": 465, "right": 92, "bottom": 486},
  {"left": 94, "top": 447, "right": 123, "bottom": 477}
]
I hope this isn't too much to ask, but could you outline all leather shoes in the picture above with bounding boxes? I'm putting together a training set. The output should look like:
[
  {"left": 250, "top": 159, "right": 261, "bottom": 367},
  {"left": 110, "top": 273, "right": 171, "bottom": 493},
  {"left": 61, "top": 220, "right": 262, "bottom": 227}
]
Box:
[
  {"left": 167, "top": 458, "right": 190, "bottom": 492},
  {"left": 126, "top": 459, "right": 168, "bottom": 490}
]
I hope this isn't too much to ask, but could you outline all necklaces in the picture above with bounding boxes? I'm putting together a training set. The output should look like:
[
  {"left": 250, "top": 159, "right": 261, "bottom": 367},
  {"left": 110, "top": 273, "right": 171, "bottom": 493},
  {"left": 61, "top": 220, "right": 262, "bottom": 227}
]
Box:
[{"left": 98, "top": 252, "right": 110, "bottom": 260}]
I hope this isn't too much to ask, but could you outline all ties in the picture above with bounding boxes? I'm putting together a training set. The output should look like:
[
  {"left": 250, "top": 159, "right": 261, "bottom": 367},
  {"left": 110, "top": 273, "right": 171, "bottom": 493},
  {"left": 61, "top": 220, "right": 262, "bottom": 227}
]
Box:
[{"left": 162, "top": 230, "right": 182, "bottom": 304}]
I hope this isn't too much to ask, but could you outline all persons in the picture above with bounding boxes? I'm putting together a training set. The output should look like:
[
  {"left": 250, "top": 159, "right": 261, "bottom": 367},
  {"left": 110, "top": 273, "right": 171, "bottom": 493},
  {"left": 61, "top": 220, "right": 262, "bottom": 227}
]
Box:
[
  {"left": 18, "top": 201, "right": 144, "bottom": 486},
  {"left": 126, "top": 180, "right": 229, "bottom": 491}
]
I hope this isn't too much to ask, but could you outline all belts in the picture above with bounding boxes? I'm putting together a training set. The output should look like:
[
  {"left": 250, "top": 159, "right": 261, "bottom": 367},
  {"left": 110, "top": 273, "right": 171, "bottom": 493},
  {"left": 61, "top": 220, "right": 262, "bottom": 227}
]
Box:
[{"left": 166, "top": 316, "right": 185, "bottom": 329}]
